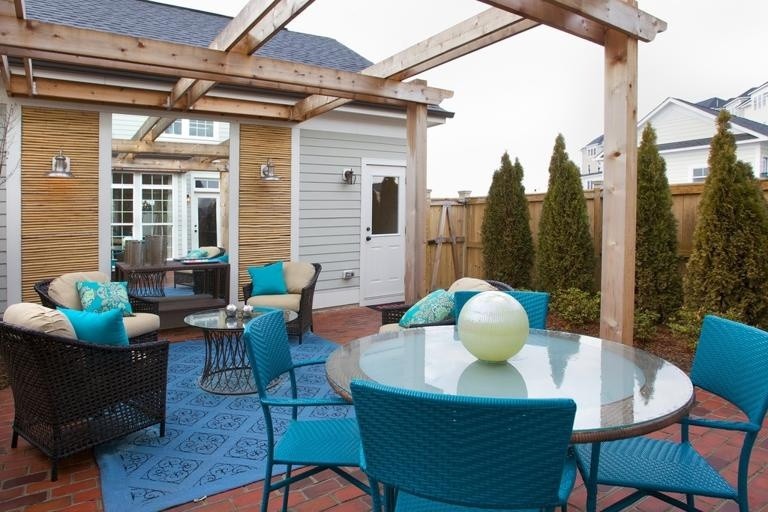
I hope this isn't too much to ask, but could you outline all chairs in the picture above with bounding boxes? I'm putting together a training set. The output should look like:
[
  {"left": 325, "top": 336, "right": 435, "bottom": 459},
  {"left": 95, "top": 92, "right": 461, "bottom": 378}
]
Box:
[
  {"left": 242, "top": 257, "right": 323, "bottom": 346},
  {"left": 1, "top": 270, "right": 172, "bottom": 480},
  {"left": 172, "top": 245, "right": 226, "bottom": 295}
]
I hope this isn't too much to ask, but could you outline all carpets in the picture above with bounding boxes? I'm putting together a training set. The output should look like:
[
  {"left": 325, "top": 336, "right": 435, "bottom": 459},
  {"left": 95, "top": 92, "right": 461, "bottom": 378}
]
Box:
[{"left": 80, "top": 329, "right": 360, "bottom": 512}]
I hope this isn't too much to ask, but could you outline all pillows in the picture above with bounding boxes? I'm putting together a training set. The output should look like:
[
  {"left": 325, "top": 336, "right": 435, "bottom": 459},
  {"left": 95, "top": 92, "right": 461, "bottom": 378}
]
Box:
[
  {"left": 181, "top": 249, "right": 209, "bottom": 259},
  {"left": 246, "top": 260, "right": 290, "bottom": 297}
]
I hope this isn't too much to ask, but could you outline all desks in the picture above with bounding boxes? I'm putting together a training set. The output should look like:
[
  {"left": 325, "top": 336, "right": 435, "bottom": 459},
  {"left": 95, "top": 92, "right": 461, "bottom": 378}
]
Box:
[{"left": 115, "top": 260, "right": 232, "bottom": 332}]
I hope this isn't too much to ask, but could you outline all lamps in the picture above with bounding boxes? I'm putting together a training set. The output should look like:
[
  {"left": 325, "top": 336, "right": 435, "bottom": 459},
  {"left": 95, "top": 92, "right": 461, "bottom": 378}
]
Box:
[
  {"left": 260, "top": 156, "right": 279, "bottom": 183},
  {"left": 342, "top": 166, "right": 357, "bottom": 188},
  {"left": 44, "top": 146, "right": 76, "bottom": 180}
]
